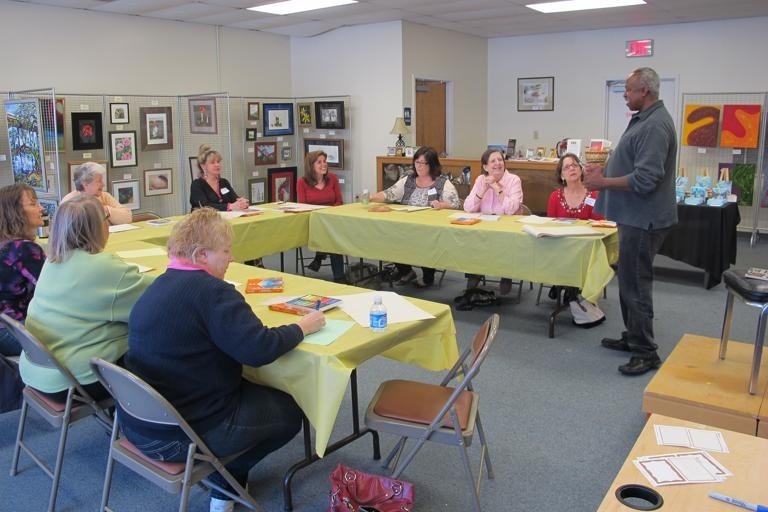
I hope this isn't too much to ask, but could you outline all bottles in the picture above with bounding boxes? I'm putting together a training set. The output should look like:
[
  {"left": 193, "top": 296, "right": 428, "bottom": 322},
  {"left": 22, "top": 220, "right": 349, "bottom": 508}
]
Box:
[{"left": 369, "top": 296, "right": 388, "bottom": 330}]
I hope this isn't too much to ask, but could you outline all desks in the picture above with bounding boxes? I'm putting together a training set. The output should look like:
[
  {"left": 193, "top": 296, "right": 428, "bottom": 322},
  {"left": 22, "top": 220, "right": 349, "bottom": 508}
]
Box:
[
  {"left": 311, "top": 203, "right": 616, "bottom": 339},
  {"left": 35, "top": 203, "right": 330, "bottom": 272},
  {"left": 590, "top": 412, "right": 766, "bottom": 511},
  {"left": 657, "top": 196, "right": 740, "bottom": 290},
  {"left": 73, "top": 241, "right": 452, "bottom": 510}
]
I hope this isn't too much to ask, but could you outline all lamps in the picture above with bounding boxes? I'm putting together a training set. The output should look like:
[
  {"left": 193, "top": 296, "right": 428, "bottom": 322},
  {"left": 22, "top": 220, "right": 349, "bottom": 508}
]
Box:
[{"left": 388, "top": 116, "right": 412, "bottom": 156}]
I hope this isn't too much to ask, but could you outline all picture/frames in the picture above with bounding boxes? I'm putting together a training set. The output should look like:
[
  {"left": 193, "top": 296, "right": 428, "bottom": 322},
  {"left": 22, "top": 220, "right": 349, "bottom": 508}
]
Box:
[
  {"left": 188, "top": 155, "right": 200, "bottom": 183},
  {"left": 2, "top": 96, "right": 174, "bottom": 215},
  {"left": 187, "top": 96, "right": 218, "bottom": 135},
  {"left": 242, "top": 98, "right": 348, "bottom": 202},
  {"left": 515, "top": 74, "right": 554, "bottom": 113}
]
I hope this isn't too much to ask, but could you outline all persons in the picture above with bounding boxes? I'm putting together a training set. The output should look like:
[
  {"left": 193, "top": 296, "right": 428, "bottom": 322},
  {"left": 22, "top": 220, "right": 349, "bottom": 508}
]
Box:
[
  {"left": 18, "top": 194, "right": 156, "bottom": 402},
  {"left": 296, "top": 150, "right": 349, "bottom": 285},
  {"left": 463, "top": 148, "right": 524, "bottom": 295},
  {"left": 581, "top": 67, "right": 679, "bottom": 375},
  {"left": 0, "top": 184, "right": 47, "bottom": 356},
  {"left": 547, "top": 152, "right": 606, "bottom": 305},
  {"left": 116, "top": 206, "right": 326, "bottom": 512},
  {"left": 368, "top": 145, "right": 462, "bottom": 286},
  {"left": 61, "top": 162, "right": 132, "bottom": 226},
  {"left": 190, "top": 144, "right": 264, "bottom": 268}
]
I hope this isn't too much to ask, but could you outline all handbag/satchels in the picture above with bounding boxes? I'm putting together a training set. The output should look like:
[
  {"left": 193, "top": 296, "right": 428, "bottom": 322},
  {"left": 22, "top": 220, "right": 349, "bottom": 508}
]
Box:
[
  {"left": 347, "top": 262, "right": 379, "bottom": 290},
  {"left": 456, "top": 289, "right": 496, "bottom": 311},
  {"left": 383, "top": 263, "right": 398, "bottom": 281},
  {"left": 328, "top": 464, "right": 414, "bottom": 512}
]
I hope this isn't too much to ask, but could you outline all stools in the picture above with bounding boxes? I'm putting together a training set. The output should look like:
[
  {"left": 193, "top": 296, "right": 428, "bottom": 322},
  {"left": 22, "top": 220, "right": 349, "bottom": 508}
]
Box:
[{"left": 721, "top": 268, "right": 766, "bottom": 395}]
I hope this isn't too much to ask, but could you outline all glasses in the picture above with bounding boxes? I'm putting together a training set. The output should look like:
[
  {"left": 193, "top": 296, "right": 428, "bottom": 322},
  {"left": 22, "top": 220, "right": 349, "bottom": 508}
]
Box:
[
  {"left": 562, "top": 163, "right": 579, "bottom": 170},
  {"left": 415, "top": 161, "right": 431, "bottom": 166}
]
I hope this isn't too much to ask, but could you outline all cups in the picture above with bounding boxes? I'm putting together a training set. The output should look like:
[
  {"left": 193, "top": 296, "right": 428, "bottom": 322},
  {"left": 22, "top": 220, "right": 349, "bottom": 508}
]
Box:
[
  {"left": 360, "top": 192, "right": 370, "bottom": 208},
  {"left": 37, "top": 214, "right": 49, "bottom": 238}
]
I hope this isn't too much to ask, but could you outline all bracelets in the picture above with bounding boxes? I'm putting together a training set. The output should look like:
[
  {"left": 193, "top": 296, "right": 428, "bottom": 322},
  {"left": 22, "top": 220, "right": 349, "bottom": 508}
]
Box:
[
  {"left": 497, "top": 190, "right": 502, "bottom": 193},
  {"left": 476, "top": 193, "right": 483, "bottom": 201}
]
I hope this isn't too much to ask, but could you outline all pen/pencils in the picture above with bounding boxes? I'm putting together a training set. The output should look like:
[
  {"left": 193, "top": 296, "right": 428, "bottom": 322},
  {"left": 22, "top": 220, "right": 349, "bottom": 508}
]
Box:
[
  {"left": 709, "top": 492, "right": 768, "bottom": 512},
  {"left": 316, "top": 300, "right": 321, "bottom": 310}
]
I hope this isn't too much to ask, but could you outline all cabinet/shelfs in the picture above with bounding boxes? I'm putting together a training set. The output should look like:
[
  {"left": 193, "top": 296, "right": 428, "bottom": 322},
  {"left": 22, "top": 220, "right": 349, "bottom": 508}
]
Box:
[{"left": 642, "top": 332, "right": 767, "bottom": 433}]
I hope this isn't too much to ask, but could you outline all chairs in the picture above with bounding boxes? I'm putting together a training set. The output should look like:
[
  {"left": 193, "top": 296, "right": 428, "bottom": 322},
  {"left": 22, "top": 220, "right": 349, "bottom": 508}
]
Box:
[
  {"left": 363, "top": 314, "right": 499, "bottom": 510},
  {"left": 2, "top": 313, "right": 117, "bottom": 512},
  {"left": 90, "top": 354, "right": 268, "bottom": 512},
  {"left": 391, "top": 200, "right": 605, "bottom": 304}
]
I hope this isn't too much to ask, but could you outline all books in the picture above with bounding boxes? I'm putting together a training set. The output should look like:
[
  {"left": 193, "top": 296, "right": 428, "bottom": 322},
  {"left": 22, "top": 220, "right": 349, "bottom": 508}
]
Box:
[
  {"left": 592, "top": 220, "right": 616, "bottom": 227},
  {"left": 223, "top": 208, "right": 261, "bottom": 219},
  {"left": 451, "top": 217, "right": 480, "bottom": 224},
  {"left": 246, "top": 277, "right": 342, "bottom": 316},
  {"left": 369, "top": 206, "right": 390, "bottom": 212},
  {"left": 148, "top": 218, "right": 176, "bottom": 225},
  {"left": 274, "top": 205, "right": 314, "bottom": 213}
]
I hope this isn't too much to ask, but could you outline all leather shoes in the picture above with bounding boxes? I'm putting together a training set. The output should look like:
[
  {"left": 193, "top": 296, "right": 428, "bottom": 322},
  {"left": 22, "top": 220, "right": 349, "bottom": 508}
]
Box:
[
  {"left": 619, "top": 354, "right": 660, "bottom": 373},
  {"left": 564, "top": 288, "right": 579, "bottom": 305},
  {"left": 602, "top": 335, "right": 631, "bottom": 351},
  {"left": 550, "top": 285, "right": 559, "bottom": 300}
]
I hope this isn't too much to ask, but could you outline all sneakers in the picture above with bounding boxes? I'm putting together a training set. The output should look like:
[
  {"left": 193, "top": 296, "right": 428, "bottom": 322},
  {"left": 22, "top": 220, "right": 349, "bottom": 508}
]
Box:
[
  {"left": 570, "top": 299, "right": 605, "bottom": 329},
  {"left": 500, "top": 278, "right": 512, "bottom": 295},
  {"left": 412, "top": 278, "right": 432, "bottom": 287},
  {"left": 309, "top": 258, "right": 321, "bottom": 271},
  {"left": 397, "top": 271, "right": 417, "bottom": 286},
  {"left": 210, "top": 482, "right": 249, "bottom": 512},
  {"left": 467, "top": 276, "right": 480, "bottom": 289}
]
